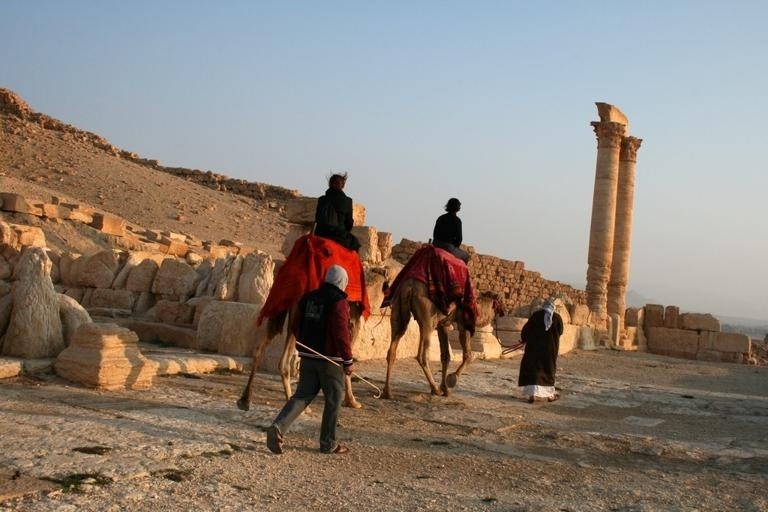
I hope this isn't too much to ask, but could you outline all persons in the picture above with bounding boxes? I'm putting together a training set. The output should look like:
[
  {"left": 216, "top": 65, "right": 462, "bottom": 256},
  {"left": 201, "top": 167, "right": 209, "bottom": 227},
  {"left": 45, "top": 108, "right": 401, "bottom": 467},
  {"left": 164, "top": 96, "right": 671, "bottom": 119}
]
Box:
[
  {"left": 432, "top": 197, "right": 471, "bottom": 266},
  {"left": 518, "top": 296, "right": 563, "bottom": 403},
  {"left": 314, "top": 170, "right": 362, "bottom": 254},
  {"left": 266, "top": 264, "right": 358, "bottom": 454}
]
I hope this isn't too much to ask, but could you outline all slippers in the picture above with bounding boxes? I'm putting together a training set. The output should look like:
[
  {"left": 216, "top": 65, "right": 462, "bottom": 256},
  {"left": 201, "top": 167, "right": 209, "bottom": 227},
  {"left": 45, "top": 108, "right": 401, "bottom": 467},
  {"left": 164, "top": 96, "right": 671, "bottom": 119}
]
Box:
[
  {"left": 266, "top": 426, "right": 284, "bottom": 454},
  {"left": 321, "top": 444, "right": 350, "bottom": 453},
  {"left": 548, "top": 395, "right": 557, "bottom": 402}
]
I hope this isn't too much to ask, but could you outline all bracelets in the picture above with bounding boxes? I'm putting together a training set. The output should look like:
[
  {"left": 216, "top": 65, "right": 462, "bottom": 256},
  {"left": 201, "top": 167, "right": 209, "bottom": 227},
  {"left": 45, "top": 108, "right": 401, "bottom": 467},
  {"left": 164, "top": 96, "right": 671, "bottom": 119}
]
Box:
[{"left": 342, "top": 358, "right": 353, "bottom": 364}]
